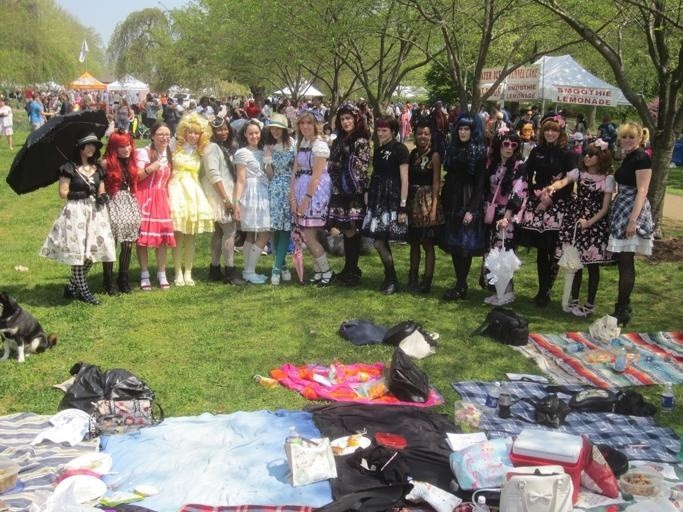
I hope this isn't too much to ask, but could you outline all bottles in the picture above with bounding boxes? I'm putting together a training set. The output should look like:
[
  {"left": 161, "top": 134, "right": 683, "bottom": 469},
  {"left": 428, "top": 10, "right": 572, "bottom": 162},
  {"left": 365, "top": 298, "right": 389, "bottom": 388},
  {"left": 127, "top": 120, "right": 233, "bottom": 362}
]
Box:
[
  {"left": 288, "top": 425, "right": 303, "bottom": 446},
  {"left": 658, "top": 380, "right": 676, "bottom": 426},
  {"left": 499, "top": 381, "right": 511, "bottom": 418},
  {"left": 608, "top": 338, "right": 640, "bottom": 374},
  {"left": 566, "top": 342, "right": 587, "bottom": 353},
  {"left": 482, "top": 381, "right": 501, "bottom": 416},
  {"left": 472, "top": 493, "right": 492, "bottom": 511}
]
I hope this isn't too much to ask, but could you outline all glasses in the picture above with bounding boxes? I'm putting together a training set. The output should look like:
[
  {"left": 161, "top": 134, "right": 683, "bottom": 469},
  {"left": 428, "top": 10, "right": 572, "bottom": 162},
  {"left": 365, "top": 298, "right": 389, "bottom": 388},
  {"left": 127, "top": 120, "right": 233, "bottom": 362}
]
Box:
[{"left": 502, "top": 141, "right": 517, "bottom": 148}]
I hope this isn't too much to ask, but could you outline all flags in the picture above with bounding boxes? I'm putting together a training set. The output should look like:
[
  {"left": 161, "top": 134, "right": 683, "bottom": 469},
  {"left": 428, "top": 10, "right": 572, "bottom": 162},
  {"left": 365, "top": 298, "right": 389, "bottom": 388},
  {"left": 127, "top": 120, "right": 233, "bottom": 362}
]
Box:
[{"left": 79, "top": 38, "right": 89, "bottom": 63}]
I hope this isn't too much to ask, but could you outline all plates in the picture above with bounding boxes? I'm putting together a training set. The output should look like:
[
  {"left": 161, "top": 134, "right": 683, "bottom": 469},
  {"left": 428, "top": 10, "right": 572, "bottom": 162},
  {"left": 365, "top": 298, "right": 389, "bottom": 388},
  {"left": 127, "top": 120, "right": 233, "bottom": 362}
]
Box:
[{"left": 327, "top": 435, "right": 370, "bottom": 454}]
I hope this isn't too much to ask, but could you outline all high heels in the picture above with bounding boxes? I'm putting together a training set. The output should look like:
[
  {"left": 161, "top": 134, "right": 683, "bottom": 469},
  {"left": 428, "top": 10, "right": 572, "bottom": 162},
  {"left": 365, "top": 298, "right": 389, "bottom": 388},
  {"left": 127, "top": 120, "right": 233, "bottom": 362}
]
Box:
[{"left": 529, "top": 290, "right": 631, "bottom": 327}]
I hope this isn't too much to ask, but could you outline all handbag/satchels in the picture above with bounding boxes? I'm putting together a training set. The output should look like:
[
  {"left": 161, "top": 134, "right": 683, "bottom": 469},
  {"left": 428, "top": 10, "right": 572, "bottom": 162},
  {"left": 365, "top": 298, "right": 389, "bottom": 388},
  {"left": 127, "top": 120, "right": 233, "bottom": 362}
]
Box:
[
  {"left": 534, "top": 387, "right": 656, "bottom": 428},
  {"left": 285, "top": 437, "right": 338, "bottom": 487},
  {"left": 391, "top": 350, "right": 428, "bottom": 403},
  {"left": 95, "top": 401, "right": 152, "bottom": 433},
  {"left": 499, "top": 465, "right": 573, "bottom": 512},
  {"left": 486, "top": 308, "right": 529, "bottom": 346}
]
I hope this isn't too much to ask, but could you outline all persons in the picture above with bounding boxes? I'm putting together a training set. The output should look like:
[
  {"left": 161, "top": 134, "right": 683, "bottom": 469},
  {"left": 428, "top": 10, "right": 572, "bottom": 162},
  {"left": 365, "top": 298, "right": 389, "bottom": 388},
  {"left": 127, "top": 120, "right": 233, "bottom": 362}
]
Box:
[
  {"left": 1, "top": 81, "right": 336, "bottom": 305},
  {"left": 325, "top": 95, "right": 654, "bottom": 329}
]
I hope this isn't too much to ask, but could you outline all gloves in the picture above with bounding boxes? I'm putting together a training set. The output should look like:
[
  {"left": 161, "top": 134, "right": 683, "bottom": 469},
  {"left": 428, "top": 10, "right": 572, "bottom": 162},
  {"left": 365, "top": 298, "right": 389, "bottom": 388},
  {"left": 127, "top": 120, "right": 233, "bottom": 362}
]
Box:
[
  {"left": 67, "top": 191, "right": 89, "bottom": 200},
  {"left": 97, "top": 192, "right": 109, "bottom": 204}
]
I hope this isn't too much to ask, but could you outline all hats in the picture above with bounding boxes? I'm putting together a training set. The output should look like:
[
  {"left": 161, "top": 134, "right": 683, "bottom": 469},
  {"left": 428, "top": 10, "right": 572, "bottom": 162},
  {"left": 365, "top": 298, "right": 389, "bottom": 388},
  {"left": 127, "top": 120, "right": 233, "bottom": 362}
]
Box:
[
  {"left": 268, "top": 114, "right": 288, "bottom": 129},
  {"left": 77, "top": 131, "right": 103, "bottom": 149}
]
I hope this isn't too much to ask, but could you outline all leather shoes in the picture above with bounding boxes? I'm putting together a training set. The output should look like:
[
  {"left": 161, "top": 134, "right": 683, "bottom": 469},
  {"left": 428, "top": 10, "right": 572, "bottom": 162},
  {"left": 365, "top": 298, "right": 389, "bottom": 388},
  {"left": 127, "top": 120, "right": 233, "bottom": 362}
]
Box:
[{"left": 380, "top": 275, "right": 517, "bottom": 306}]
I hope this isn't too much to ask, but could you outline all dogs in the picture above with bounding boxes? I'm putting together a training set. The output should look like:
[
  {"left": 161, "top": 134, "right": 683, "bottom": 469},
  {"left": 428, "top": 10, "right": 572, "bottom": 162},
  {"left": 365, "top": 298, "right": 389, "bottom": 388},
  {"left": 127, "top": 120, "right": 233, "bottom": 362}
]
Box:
[{"left": 0, "top": 291, "right": 57, "bottom": 363}]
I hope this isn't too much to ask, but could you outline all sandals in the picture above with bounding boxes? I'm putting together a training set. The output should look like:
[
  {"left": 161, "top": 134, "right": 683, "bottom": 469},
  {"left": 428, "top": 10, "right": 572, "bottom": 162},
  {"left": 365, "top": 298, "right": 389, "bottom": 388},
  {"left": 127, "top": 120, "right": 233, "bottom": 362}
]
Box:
[
  {"left": 64, "top": 271, "right": 195, "bottom": 305},
  {"left": 210, "top": 263, "right": 362, "bottom": 287}
]
